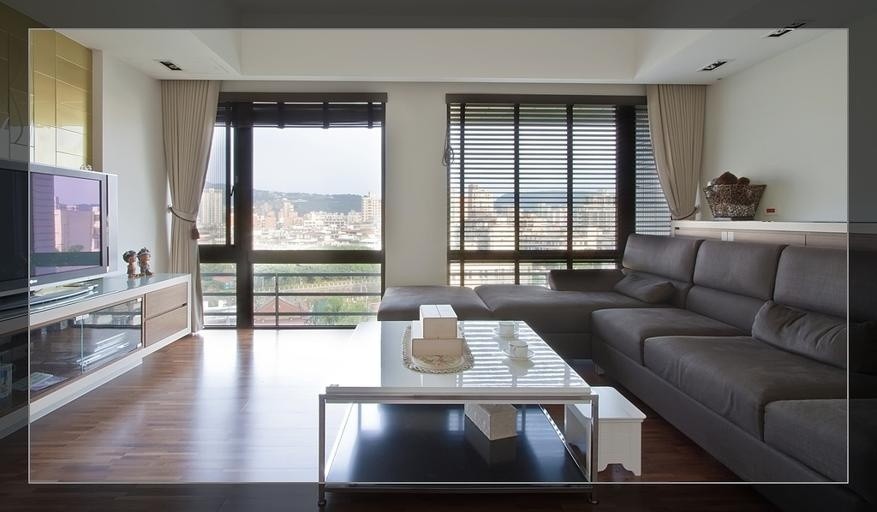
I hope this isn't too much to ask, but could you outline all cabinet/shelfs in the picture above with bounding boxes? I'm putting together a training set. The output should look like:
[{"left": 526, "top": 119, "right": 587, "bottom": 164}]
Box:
[
  {"left": 0, "top": 272, "right": 191, "bottom": 440},
  {"left": 669, "top": 219, "right": 876, "bottom": 251}
]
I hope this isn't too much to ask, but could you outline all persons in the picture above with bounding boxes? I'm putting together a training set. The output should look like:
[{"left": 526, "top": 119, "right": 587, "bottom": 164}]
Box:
[
  {"left": 120, "top": 250, "right": 138, "bottom": 280},
  {"left": 137, "top": 247, "right": 152, "bottom": 277}
]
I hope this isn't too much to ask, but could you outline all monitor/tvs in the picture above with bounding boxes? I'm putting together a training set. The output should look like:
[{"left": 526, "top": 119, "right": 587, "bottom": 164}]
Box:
[{"left": 30, "top": 162, "right": 120, "bottom": 307}]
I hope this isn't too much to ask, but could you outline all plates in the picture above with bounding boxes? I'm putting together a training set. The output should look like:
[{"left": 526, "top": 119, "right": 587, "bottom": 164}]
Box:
[
  {"left": 502, "top": 347, "right": 535, "bottom": 362},
  {"left": 493, "top": 326, "right": 519, "bottom": 339},
  {"left": 502, "top": 357, "right": 535, "bottom": 368}
]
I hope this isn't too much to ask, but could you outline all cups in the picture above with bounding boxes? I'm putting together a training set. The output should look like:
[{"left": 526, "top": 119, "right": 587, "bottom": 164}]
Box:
[
  {"left": 508, "top": 365, "right": 529, "bottom": 377},
  {"left": 498, "top": 321, "right": 514, "bottom": 334},
  {"left": 509, "top": 340, "right": 529, "bottom": 358}
]
